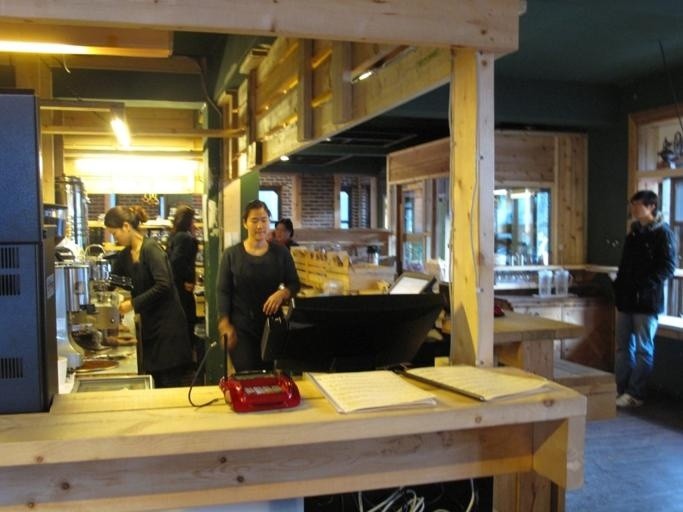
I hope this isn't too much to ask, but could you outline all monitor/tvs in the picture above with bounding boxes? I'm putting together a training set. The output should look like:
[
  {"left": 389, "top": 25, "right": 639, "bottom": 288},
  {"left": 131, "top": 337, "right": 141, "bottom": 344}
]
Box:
[
  {"left": 274, "top": 293, "right": 447, "bottom": 371},
  {"left": 386, "top": 271, "right": 433, "bottom": 295}
]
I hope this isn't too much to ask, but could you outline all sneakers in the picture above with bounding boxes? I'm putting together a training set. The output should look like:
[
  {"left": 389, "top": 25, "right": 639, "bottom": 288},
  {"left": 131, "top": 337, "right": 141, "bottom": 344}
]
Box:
[{"left": 615, "top": 392, "right": 647, "bottom": 409}]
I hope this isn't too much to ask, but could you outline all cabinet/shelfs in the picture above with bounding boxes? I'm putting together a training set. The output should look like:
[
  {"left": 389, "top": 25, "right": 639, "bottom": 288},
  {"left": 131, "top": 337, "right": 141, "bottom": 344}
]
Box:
[
  {"left": 495, "top": 294, "right": 617, "bottom": 373},
  {"left": 626, "top": 102, "right": 683, "bottom": 406}
]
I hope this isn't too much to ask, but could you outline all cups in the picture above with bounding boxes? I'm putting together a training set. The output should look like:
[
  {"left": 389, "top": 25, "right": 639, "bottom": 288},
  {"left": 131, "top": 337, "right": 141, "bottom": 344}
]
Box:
[{"left": 539, "top": 271, "right": 573, "bottom": 297}]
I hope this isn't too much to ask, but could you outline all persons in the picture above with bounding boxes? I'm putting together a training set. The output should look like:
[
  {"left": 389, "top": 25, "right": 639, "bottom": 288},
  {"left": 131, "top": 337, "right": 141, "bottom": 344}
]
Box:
[
  {"left": 104, "top": 205, "right": 200, "bottom": 382},
  {"left": 612, "top": 191, "right": 677, "bottom": 409},
  {"left": 215, "top": 200, "right": 301, "bottom": 370}
]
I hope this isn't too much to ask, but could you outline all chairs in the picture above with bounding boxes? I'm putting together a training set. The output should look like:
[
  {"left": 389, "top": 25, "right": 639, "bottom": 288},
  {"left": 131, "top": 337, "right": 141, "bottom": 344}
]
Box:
[{"left": 88, "top": 220, "right": 172, "bottom": 260}]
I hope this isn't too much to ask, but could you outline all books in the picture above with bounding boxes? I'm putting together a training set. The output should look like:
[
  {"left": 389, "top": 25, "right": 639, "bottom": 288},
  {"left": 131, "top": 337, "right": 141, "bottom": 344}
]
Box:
[
  {"left": 405, "top": 364, "right": 552, "bottom": 402},
  {"left": 307, "top": 370, "right": 436, "bottom": 415}
]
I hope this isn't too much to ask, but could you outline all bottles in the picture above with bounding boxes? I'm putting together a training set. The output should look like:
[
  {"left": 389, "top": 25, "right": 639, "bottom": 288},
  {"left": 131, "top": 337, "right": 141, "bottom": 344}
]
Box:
[{"left": 367, "top": 245, "right": 379, "bottom": 263}]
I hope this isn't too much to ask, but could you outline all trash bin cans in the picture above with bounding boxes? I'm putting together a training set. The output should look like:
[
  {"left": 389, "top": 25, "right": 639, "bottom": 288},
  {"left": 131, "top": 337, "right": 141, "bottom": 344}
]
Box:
[{"left": 194, "top": 323, "right": 205, "bottom": 370}]
